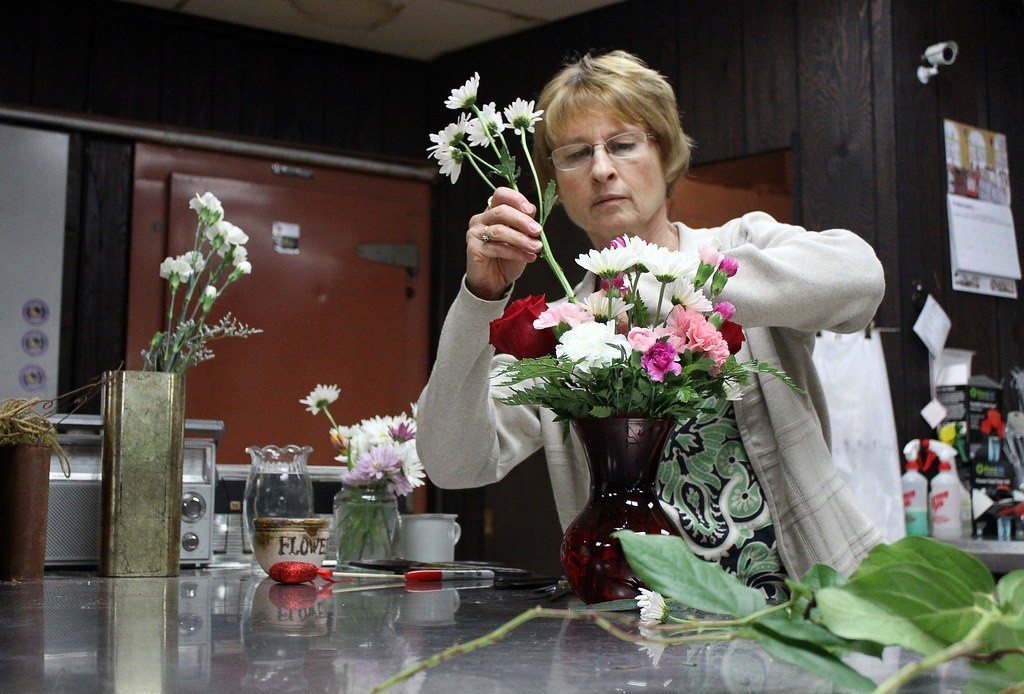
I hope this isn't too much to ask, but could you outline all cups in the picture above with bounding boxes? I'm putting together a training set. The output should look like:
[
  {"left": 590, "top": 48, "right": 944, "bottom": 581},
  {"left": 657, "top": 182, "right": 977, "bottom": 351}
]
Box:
[
  {"left": 399, "top": 514, "right": 461, "bottom": 562},
  {"left": 252, "top": 517, "right": 330, "bottom": 583}
]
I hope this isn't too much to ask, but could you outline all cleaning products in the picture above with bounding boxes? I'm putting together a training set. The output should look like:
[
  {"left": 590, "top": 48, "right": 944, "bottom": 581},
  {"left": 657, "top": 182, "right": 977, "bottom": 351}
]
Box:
[
  {"left": 900, "top": 438, "right": 927, "bottom": 535},
  {"left": 922, "top": 438, "right": 962, "bottom": 543}
]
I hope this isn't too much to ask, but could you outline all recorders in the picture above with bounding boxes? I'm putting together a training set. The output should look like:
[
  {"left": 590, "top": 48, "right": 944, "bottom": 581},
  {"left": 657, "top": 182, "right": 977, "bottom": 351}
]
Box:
[{"left": 44, "top": 412, "right": 225, "bottom": 568}]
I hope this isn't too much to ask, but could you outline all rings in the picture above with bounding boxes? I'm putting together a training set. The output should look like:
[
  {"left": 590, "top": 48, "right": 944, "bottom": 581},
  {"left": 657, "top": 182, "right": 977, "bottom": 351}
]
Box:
[
  {"left": 482, "top": 225, "right": 489, "bottom": 243},
  {"left": 487, "top": 195, "right": 493, "bottom": 208}
]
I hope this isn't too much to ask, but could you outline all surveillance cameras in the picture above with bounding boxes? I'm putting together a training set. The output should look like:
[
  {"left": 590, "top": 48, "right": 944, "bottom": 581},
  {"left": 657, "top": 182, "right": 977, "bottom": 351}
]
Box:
[{"left": 924, "top": 41, "right": 958, "bottom": 66}]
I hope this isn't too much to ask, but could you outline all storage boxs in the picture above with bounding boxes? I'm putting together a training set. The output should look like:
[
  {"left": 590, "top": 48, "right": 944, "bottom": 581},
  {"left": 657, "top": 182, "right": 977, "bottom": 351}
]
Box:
[{"left": 929, "top": 349, "right": 1012, "bottom": 535}]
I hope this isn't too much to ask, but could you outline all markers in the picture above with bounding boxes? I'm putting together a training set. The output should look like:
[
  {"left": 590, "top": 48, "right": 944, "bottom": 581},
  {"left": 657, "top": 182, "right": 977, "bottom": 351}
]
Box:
[
  {"left": 405, "top": 569, "right": 496, "bottom": 581},
  {"left": 403, "top": 581, "right": 495, "bottom": 592}
]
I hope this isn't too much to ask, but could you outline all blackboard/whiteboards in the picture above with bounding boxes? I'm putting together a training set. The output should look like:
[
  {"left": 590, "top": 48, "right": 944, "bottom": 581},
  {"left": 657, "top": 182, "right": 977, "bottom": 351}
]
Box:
[{"left": 0, "top": 123, "right": 73, "bottom": 414}]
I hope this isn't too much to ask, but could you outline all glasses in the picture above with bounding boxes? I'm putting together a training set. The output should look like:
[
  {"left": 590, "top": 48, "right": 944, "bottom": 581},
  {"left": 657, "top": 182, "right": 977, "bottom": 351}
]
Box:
[{"left": 546, "top": 130, "right": 656, "bottom": 172}]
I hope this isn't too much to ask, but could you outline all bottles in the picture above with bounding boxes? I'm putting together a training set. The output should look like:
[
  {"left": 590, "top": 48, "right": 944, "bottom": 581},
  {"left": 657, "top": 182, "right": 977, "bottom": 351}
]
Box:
[{"left": 243, "top": 445, "right": 314, "bottom": 556}]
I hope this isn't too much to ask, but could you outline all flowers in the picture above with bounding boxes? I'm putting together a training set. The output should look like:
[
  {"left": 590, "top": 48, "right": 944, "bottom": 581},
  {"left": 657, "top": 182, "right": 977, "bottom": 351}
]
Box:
[
  {"left": 143, "top": 191, "right": 264, "bottom": 373},
  {"left": 298, "top": 384, "right": 426, "bottom": 558},
  {"left": 426, "top": 71, "right": 580, "bottom": 302},
  {"left": 637, "top": 629, "right": 758, "bottom": 668},
  {"left": 490, "top": 234, "right": 805, "bottom": 445},
  {"left": 636, "top": 588, "right": 795, "bottom": 628}
]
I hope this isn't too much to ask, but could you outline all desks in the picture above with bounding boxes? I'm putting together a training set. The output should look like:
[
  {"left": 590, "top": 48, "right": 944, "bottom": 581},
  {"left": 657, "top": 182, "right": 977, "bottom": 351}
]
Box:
[{"left": 0, "top": 552, "right": 1023, "bottom": 694}]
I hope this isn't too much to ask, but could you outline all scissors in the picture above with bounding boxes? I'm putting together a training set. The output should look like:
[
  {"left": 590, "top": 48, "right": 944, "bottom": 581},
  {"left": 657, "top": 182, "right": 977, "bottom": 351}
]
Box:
[
  {"left": 348, "top": 557, "right": 538, "bottom": 581},
  {"left": 521, "top": 574, "right": 574, "bottom": 605}
]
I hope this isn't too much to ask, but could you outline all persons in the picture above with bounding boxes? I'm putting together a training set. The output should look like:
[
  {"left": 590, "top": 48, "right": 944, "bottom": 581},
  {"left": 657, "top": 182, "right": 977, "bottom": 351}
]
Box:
[{"left": 415, "top": 49, "right": 887, "bottom": 609}]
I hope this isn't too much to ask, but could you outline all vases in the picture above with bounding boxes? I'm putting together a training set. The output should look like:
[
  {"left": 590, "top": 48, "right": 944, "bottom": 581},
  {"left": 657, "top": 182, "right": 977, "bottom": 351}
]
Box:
[
  {"left": 238, "top": 580, "right": 309, "bottom": 693},
  {"left": 333, "top": 483, "right": 401, "bottom": 570},
  {"left": 100, "top": 371, "right": 187, "bottom": 577},
  {"left": 245, "top": 445, "right": 314, "bottom": 578},
  {"left": 559, "top": 420, "right": 683, "bottom": 605},
  {"left": 100, "top": 575, "right": 180, "bottom": 692}
]
